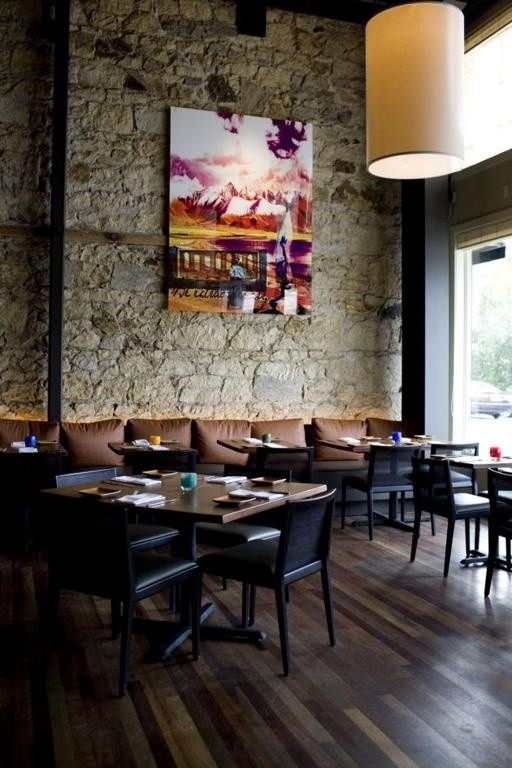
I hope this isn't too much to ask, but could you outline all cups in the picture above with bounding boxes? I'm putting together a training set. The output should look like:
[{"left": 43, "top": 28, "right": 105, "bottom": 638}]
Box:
[
  {"left": 391, "top": 431, "right": 402, "bottom": 441},
  {"left": 26, "top": 434, "right": 38, "bottom": 448},
  {"left": 261, "top": 433, "right": 273, "bottom": 444},
  {"left": 488, "top": 447, "right": 502, "bottom": 460},
  {"left": 149, "top": 435, "right": 160, "bottom": 446},
  {"left": 181, "top": 472, "right": 198, "bottom": 492}
]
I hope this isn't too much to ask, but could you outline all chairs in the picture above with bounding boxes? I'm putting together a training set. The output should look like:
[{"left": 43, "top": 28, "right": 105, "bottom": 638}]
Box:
[
  {"left": 39, "top": 493, "right": 204, "bottom": 697},
  {"left": 483, "top": 463, "right": 512, "bottom": 599},
  {"left": 339, "top": 444, "right": 423, "bottom": 540},
  {"left": 53, "top": 466, "right": 184, "bottom": 642},
  {"left": 197, "top": 487, "right": 337, "bottom": 675},
  {"left": 196, "top": 464, "right": 293, "bottom": 629},
  {"left": 404, "top": 453, "right": 492, "bottom": 579},
  {"left": 403, "top": 443, "right": 480, "bottom": 537}
]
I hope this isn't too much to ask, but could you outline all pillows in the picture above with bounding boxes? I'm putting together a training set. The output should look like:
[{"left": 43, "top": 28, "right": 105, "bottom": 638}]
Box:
[
  {"left": 309, "top": 417, "right": 368, "bottom": 462},
  {"left": 191, "top": 418, "right": 252, "bottom": 465},
  {"left": 56, "top": 420, "right": 128, "bottom": 469},
  {"left": 126, "top": 416, "right": 192, "bottom": 463},
  {"left": 248, "top": 418, "right": 310, "bottom": 462},
  {"left": 0, "top": 419, "right": 62, "bottom": 454},
  {"left": 363, "top": 418, "right": 410, "bottom": 459}
]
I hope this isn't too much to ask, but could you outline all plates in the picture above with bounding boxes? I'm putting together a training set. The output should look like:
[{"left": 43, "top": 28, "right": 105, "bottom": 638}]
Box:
[
  {"left": 215, "top": 493, "right": 254, "bottom": 507},
  {"left": 361, "top": 434, "right": 433, "bottom": 446},
  {"left": 251, "top": 475, "right": 287, "bottom": 486},
  {"left": 142, "top": 469, "right": 178, "bottom": 480},
  {"left": 121, "top": 439, "right": 180, "bottom": 450},
  {"left": 36, "top": 439, "right": 60, "bottom": 444},
  {"left": 81, "top": 486, "right": 121, "bottom": 498}
]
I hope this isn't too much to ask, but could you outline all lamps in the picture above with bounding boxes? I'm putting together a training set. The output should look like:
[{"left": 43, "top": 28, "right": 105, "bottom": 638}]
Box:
[{"left": 366, "top": 0, "right": 467, "bottom": 180}]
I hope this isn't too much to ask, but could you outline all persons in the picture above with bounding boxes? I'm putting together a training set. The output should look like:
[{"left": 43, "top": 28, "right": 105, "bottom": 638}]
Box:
[{"left": 227, "top": 255, "right": 245, "bottom": 313}]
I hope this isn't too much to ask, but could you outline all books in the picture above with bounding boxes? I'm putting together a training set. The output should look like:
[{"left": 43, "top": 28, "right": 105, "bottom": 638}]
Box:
[
  {"left": 150, "top": 445, "right": 170, "bottom": 450},
  {"left": 111, "top": 475, "right": 166, "bottom": 505},
  {"left": 243, "top": 438, "right": 288, "bottom": 448},
  {"left": 339, "top": 437, "right": 423, "bottom": 447},
  {"left": 205, "top": 476, "right": 284, "bottom": 500}
]
